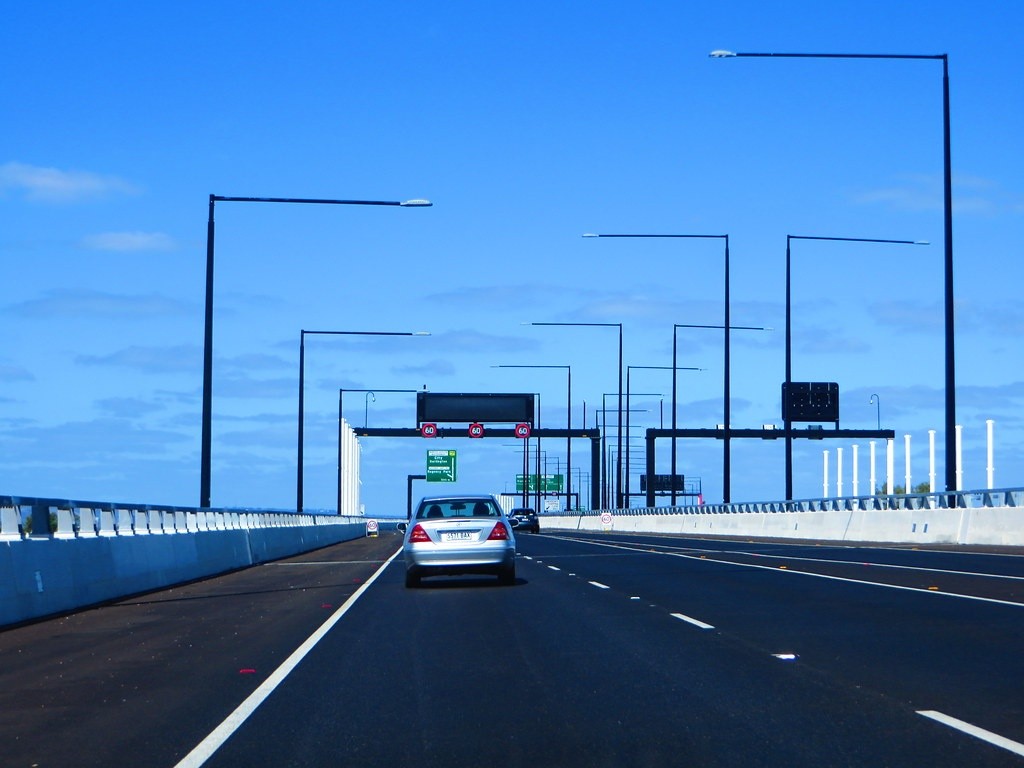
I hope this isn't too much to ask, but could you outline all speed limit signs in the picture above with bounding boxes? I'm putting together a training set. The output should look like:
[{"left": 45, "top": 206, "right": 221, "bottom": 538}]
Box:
[
  {"left": 469, "top": 423, "right": 483, "bottom": 438},
  {"left": 516, "top": 423, "right": 531, "bottom": 439},
  {"left": 366, "top": 519, "right": 377, "bottom": 532},
  {"left": 601, "top": 513, "right": 611, "bottom": 524},
  {"left": 422, "top": 423, "right": 436, "bottom": 438}
]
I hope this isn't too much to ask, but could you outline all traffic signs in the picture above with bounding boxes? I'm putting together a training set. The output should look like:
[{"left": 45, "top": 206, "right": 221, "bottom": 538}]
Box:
[
  {"left": 515, "top": 474, "right": 563, "bottom": 491},
  {"left": 426, "top": 449, "right": 458, "bottom": 483}
]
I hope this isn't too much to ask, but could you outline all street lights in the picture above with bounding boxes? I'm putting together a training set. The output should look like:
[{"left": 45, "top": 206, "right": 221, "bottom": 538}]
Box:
[
  {"left": 706, "top": 48, "right": 959, "bottom": 512},
  {"left": 491, "top": 322, "right": 708, "bottom": 510},
  {"left": 337, "top": 388, "right": 430, "bottom": 518},
  {"left": 785, "top": 233, "right": 933, "bottom": 511},
  {"left": 295, "top": 330, "right": 430, "bottom": 513},
  {"left": 582, "top": 231, "right": 733, "bottom": 504},
  {"left": 672, "top": 323, "right": 774, "bottom": 510},
  {"left": 199, "top": 195, "right": 434, "bottom": 516}
]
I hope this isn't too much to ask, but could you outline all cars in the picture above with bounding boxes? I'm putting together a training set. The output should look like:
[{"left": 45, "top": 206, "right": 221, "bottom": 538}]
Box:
[
  {"left": 506, "top": 507, "right": 540, "bottom": 535},
  {"left": 393, "top": 492, "right": 520, "bottom": 587}
]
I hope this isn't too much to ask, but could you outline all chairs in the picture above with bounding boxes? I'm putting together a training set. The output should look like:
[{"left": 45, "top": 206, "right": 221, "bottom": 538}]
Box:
[
  {"left": 473, "top": 504, "right": 490, "bottom": 517},
  {"left": 427, "top": 505, "right": 444, "bottom": 518}
]
